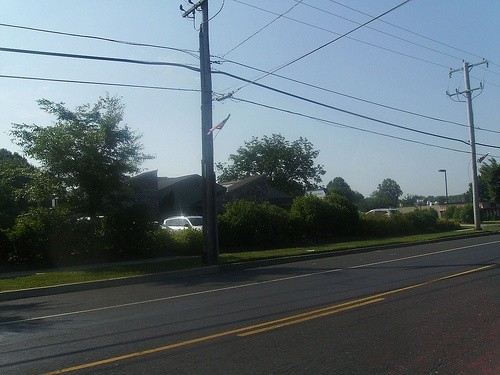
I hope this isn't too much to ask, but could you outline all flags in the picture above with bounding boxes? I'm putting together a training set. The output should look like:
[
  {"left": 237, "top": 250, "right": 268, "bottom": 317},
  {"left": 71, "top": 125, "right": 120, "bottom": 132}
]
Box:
[
  {"left": 206, "top": 116, "right": 229, "bottom": 136},
  {"left": 477, "top": 153, "right": 488, "bottom": 164}
]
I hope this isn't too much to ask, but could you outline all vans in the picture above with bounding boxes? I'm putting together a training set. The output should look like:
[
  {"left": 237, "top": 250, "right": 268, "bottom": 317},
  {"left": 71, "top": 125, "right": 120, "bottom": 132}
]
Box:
[{"left": 163, "top": 216, "right": 203, "bottom": 230}]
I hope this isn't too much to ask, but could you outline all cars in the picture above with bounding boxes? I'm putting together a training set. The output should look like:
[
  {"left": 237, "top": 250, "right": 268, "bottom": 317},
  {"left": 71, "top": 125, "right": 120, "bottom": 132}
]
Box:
[{"left": 365, "top": 209, "right": 402, "bottom": 218}]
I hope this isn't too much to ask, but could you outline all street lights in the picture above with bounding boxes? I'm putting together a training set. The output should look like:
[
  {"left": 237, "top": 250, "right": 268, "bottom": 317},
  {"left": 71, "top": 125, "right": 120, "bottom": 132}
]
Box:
[{"left": 438, "top": 169, "right": 449, "bottom": 204}]
